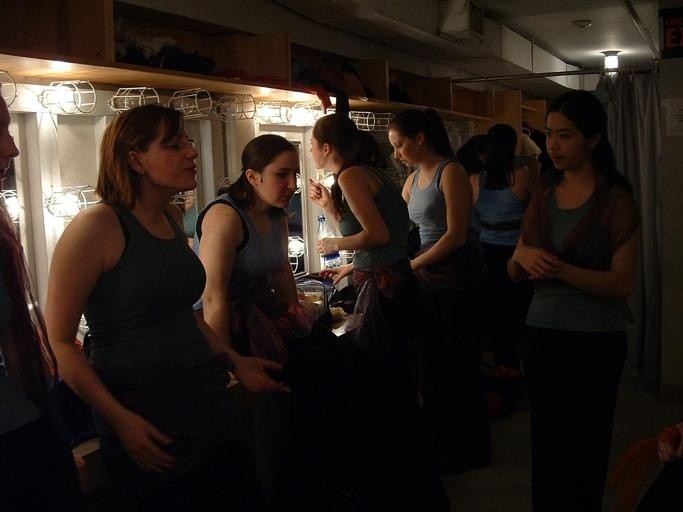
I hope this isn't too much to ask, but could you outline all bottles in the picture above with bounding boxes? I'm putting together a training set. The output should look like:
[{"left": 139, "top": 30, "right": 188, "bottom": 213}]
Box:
[{"left": 316, "top": 213, "right": 344, "bottom": 270}]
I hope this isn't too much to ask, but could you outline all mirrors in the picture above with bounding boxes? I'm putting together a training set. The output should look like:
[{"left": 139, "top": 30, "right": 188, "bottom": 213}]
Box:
[{"left": 267, "top": 140, "right": 308, "bottom": 279}]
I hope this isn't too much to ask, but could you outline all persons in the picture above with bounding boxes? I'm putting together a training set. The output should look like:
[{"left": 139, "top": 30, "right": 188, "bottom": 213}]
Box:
[
  {"left": 45, "top": 104, "right": 264, "bottom": 510},
  {"left": 506, "top": 88, "right": 637, "bottom": 510},
  {"left": 307, "top": 105, "right": 543, "bottom": 509},
  {"left": 1, "top": 95, "right": 80, "bottom": 511},
  {"left": 194, "top": 134, "right": 376, "bottom": 510}
]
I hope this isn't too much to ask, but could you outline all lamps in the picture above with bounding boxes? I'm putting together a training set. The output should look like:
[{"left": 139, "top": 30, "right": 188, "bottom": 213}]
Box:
[
  {"left": 286, "top": 235, "right": 307, "bottom": 259},
  {"left": 599, "top": 48, "right": 621, "bottom": 80}
]
